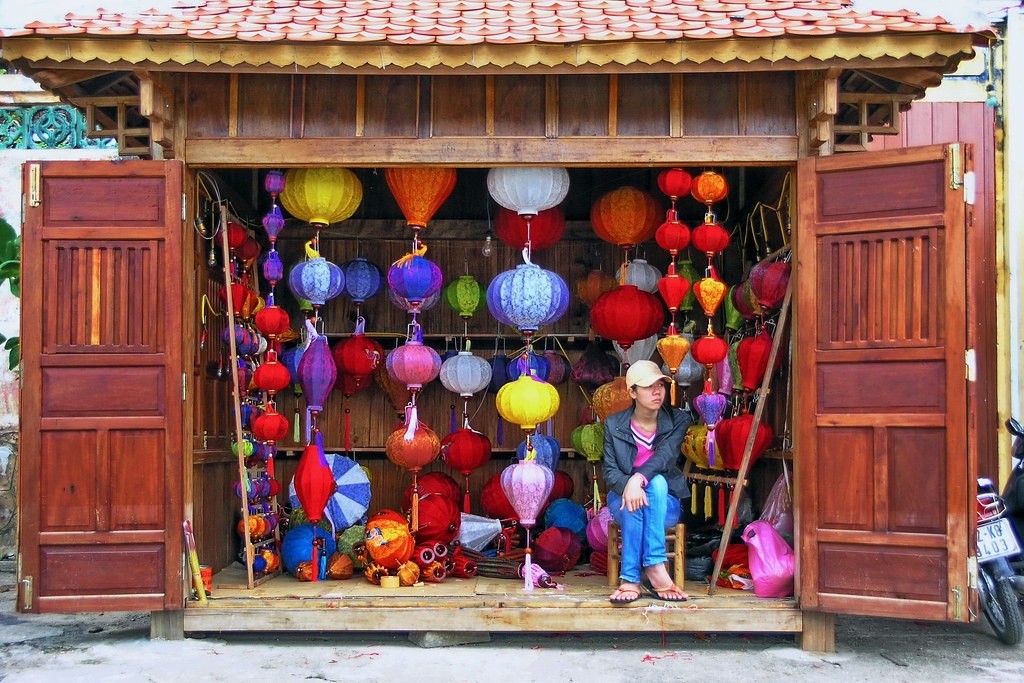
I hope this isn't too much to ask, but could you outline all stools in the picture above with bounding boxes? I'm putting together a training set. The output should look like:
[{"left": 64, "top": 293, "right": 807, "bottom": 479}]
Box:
[{"left": 607, "top": 519, "right": 685, "bottom": 592}]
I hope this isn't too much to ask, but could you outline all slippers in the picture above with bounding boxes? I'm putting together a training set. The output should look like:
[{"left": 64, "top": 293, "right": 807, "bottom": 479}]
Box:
[
  {"left": 643, "top": 579, "right": 687, "bottom": 601},
  {"left": 609, "top": 588, "right": 642, "bottom": 603}
]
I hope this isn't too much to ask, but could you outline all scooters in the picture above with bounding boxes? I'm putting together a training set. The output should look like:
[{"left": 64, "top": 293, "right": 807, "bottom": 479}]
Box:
[{"left": 977, "top": 415, "right": 1024, "bottom": 645}]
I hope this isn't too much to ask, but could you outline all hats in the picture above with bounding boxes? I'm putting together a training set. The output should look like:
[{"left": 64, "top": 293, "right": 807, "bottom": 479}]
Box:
[{"left": 626, "top": 360, "right": 672, "bottom": 390}]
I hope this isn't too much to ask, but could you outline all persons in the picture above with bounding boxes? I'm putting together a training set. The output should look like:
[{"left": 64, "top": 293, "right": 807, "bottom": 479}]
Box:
[{"left": 597, "top": 360, "right": 694, "bottom": 601}]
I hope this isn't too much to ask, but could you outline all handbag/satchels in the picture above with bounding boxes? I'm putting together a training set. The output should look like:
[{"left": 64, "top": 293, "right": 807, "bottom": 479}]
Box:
[
  {"left": 742, "top": 520, "right": 795, "bottom": 596},
  {"left": 759, "top": 471, "right": 794, "bottom": 543}
]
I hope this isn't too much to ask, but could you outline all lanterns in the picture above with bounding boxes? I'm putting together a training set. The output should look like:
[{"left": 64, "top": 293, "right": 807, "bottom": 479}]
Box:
[{"left": 218, "top": 164, "right": 789, "bottom": 582}]
